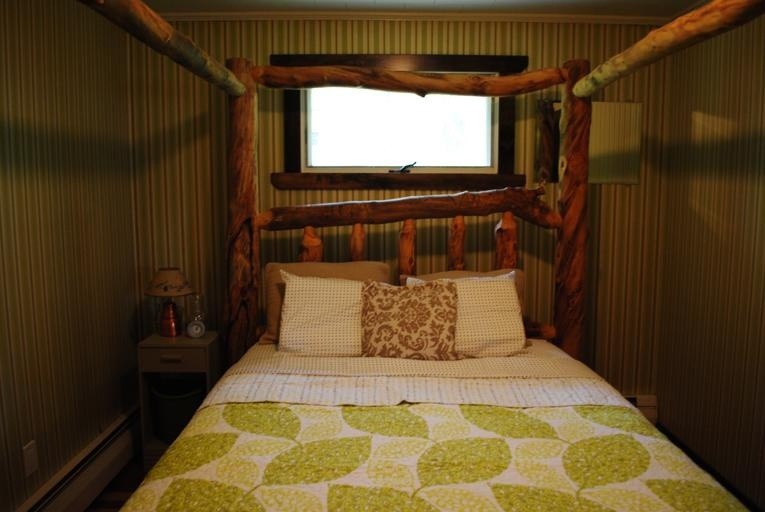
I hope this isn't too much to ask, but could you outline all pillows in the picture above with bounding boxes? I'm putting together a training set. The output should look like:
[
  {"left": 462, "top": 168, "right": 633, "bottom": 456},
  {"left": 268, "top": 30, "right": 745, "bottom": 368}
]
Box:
[{"left": 258, "top": 261, "right": 533, "bottom": 361}]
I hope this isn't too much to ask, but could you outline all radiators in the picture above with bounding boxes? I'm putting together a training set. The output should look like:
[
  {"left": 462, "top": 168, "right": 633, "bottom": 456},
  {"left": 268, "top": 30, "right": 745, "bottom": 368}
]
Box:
[{"left": 554, "top": 101, "right": 643, "bottom": 185}]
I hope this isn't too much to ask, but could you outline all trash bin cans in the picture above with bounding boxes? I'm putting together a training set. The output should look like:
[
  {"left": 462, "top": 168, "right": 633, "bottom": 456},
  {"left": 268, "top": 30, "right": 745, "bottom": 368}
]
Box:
[{"left": 149, "top": 373, "right": 205, "bottom": 443}]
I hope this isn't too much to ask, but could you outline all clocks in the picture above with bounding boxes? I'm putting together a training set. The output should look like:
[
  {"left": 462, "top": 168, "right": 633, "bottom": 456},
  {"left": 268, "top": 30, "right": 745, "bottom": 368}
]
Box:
[{"left": 186, "top": 321, "right": 206, "bottom": 340}]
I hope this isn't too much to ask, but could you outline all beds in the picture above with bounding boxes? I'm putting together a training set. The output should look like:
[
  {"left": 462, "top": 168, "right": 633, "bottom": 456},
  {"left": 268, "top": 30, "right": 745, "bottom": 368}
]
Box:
[{"left": 116, "top": 327, "right": 764, "bottom": 512}]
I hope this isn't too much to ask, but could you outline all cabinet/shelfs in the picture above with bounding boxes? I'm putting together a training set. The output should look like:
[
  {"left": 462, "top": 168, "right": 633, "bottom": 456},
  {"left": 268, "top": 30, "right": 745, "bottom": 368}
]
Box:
[{"left": 137, "top": 330, "right": 220, "bottom": 466}]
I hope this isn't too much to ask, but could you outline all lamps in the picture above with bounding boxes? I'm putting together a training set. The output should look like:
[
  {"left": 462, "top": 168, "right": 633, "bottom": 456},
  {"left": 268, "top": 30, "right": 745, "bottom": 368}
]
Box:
[{"left": 144, "top": 267, "right": 193, "bottom": 338}]
